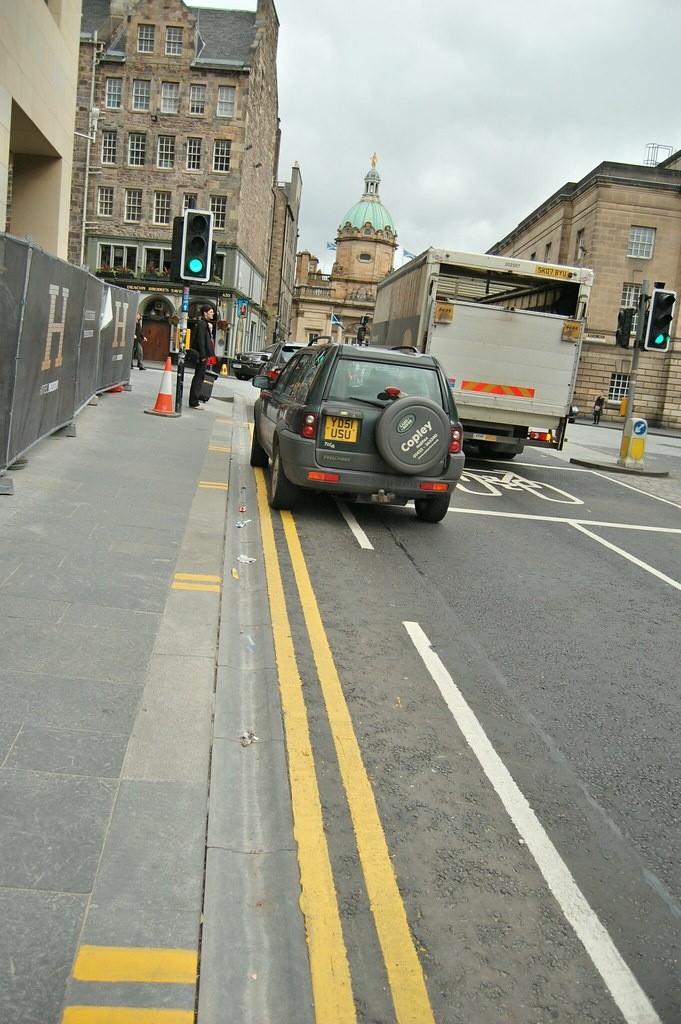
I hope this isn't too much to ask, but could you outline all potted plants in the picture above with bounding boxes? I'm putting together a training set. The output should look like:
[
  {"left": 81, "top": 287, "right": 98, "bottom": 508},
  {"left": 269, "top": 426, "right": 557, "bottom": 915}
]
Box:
[
  {"left": 142, "top": 263, "right": 170, "bottom": 281},
  {"left": 115, "top": 265, "right": 140, "bottom": 280},
  {"left": 94, "top": 264, "right": 116, "bottom": 277}
]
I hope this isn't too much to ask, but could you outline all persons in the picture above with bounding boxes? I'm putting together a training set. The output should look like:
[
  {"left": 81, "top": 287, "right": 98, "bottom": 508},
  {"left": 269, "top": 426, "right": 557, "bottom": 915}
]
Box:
[
  {"left": 188, "top": 305, "right": 214, "bottom": 410},
  {"left": 593, "top": 392, "right": 604, "bottom": 424},
  {"left": 130, "top": 312, "right": 147, "bottom": 370}
]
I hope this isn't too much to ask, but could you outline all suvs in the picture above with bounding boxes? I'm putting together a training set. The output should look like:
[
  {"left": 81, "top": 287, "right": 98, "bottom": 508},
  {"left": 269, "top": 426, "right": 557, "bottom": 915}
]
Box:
[
  {"left": 249, "top": 333, "right": 468, "bottom": 524},
  {"left": 232, "top": 340, "right": 321, "bottom": 392}
]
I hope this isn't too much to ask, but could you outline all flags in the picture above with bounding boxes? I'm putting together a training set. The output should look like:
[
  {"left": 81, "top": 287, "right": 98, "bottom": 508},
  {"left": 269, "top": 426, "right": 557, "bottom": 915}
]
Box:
[{"left": 330, "top": 314, "right": 344, "bottom": 329}]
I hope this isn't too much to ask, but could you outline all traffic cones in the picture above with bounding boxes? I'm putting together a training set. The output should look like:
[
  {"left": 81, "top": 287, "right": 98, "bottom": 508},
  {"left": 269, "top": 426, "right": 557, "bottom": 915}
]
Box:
[{"left": 144, "top": 356, "right": 182, "bottom": 418}]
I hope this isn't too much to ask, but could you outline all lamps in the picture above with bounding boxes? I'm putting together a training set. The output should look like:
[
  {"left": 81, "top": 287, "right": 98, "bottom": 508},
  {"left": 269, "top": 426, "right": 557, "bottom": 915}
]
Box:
[
  {"left": 151, "top": 115, "right": 157, "bottom": 122},
  {"left": 580, "top": 246, "right": 587, "bottom": 252},
  {"left": 245, "top": 144, "right": 253, "bottom": 150},
  {"left": 254, "top": 162, "right": 262, "bottom": 168}
]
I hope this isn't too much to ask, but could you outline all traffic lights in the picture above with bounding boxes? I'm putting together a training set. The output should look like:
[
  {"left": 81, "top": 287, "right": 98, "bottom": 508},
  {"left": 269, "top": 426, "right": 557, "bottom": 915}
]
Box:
[
  {"left": 644, "top": 288, "right": 677, "bottom": 352},
  {"left": 616, "top": 309, "right": 634, "bottom": 347},
  {"left": 179, "top": 208, "right": 214, "bottom": 283}
]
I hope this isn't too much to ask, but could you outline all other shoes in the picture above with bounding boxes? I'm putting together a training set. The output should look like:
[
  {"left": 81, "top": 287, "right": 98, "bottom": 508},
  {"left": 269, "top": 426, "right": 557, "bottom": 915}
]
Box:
[
  {"left": 131, "top": 364, "right": 133, "bottom": 369},
  {"left": 191, "top": 404, "right": 204, "bottom": 409},
  {"left": 139, "top": 366, "right": 146, "bottom": 370}
]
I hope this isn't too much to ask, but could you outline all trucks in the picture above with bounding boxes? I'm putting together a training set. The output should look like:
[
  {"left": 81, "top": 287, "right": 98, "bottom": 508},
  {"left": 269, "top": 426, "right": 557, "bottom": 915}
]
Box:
[{"left": 356, "top": 246, "right": 594, "bottom": 459}]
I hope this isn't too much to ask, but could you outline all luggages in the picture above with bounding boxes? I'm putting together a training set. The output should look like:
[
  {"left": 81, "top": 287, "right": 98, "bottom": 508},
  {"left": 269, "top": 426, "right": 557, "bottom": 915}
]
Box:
[{"left": 198, "top": 363, "right": 215, "bottom": 403}]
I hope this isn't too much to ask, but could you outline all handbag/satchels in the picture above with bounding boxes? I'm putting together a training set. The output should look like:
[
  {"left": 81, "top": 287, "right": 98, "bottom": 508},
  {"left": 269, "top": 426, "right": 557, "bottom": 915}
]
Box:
[
  {"left": 206, "top": 356, "right": 216, "bottom": 365},
  {"left": 595, "top": 406, "right": 600, "bottom": 411},
  {"left": 572, "top": 406, "right": 579, "bottom": 413},
  {"left": 187, "top": 348, "right": 200, "bottom": 363}
]
